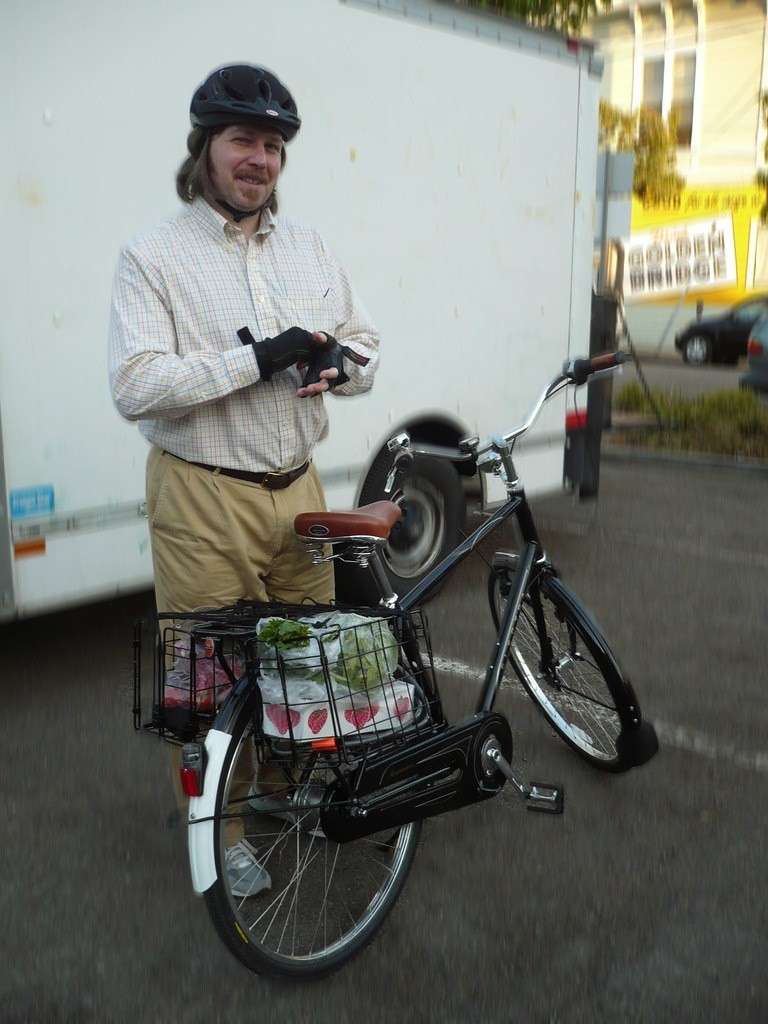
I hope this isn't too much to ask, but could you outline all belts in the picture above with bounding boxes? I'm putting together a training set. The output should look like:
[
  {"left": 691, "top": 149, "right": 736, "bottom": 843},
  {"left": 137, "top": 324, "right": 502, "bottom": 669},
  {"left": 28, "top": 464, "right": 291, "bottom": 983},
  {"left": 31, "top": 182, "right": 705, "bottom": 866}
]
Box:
[{"left": 164, "top": 450, "right": 313, "bottom": 490}]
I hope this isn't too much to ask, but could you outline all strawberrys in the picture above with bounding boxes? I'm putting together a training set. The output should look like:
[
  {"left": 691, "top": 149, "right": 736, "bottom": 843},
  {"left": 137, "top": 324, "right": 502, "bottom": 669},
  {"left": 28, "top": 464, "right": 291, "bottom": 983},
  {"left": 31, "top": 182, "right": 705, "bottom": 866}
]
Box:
[
  {"left": 308, "top": 708, "right": 328, "bottom": 733},
  {"left": 265, "top": 705, "right": 300, "bottom": 735},
  {"left": 394, "top": 698, "right": 409, "bottom": 720},
  {"left": 344, "top": 704, "right": 380, "bottom": 730}
]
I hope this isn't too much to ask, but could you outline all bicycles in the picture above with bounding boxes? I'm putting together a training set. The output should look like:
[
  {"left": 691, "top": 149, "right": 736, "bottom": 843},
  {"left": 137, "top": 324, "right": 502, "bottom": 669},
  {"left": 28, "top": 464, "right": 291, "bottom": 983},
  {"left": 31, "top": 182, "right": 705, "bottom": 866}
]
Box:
[{"left": 131, "top": 347, "right": 659, "bottom": 977}]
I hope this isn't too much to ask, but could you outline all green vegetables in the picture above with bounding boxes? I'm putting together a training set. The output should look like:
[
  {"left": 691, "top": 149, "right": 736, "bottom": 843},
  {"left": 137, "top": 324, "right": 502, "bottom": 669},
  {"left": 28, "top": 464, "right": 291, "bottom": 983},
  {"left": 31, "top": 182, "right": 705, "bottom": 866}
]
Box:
[{"left": 253, "top": 614, "right": 398, "bottom": 689}]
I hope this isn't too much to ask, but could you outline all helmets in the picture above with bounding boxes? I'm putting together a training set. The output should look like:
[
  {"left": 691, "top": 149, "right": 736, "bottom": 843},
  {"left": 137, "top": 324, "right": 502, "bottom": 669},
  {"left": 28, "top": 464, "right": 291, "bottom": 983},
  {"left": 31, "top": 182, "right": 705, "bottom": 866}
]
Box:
[{"left": 190, "top": 65, "right": 301, "bottom": 142}]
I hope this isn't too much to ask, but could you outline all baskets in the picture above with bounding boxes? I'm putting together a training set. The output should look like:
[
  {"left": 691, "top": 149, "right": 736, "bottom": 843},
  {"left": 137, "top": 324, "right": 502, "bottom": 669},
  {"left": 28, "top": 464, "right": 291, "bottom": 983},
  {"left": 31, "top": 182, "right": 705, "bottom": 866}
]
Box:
[{"left": 131, "top": 597, "right": 445, "bottom": 770}]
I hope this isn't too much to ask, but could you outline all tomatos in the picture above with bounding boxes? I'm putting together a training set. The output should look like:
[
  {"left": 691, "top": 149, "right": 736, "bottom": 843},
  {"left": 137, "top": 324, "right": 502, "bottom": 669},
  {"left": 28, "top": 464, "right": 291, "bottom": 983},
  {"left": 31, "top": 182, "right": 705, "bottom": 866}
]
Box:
[{"left": 165, "top": 641, "right": 241, "bottom": 709}]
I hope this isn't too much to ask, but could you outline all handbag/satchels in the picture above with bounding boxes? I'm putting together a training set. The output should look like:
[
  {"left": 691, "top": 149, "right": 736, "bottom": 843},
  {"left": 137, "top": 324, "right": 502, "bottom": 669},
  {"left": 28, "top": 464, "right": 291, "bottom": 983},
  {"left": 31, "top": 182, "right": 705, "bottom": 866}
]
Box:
[
  {"left": 163, "top": 632, "right": 246, "bottom": 710},
  {"left": 256, "top": 610, "right": 398, "bottom": 713}
]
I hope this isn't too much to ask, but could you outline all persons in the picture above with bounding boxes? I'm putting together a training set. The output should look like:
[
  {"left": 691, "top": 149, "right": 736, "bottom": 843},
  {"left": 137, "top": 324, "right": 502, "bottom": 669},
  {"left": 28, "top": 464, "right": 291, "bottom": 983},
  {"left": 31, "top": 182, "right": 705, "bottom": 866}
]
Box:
[{"left": 110, "top": 64, "right": 383, "bottom": 901}]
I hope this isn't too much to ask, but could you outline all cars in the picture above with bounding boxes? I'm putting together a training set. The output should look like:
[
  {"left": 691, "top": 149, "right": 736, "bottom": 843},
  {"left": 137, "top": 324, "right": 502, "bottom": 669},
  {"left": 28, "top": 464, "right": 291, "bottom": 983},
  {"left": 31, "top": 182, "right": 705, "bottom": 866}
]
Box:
[
  {"left": 738, "top": 307, "right": 768, "bottom": 397},
  {"left": 675, "top": 290, "right": 768, "bottom": 368}
]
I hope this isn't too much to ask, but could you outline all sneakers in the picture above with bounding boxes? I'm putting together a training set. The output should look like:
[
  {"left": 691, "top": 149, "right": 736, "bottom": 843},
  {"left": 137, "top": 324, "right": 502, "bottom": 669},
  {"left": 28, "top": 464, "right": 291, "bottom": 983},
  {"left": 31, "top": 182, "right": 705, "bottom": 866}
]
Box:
[
  {"left": 247, "top": 783, "right": 327, "bottom": 837},
  {"left": 223, "top": 837, "right": 271, "bottom": 897}
]
